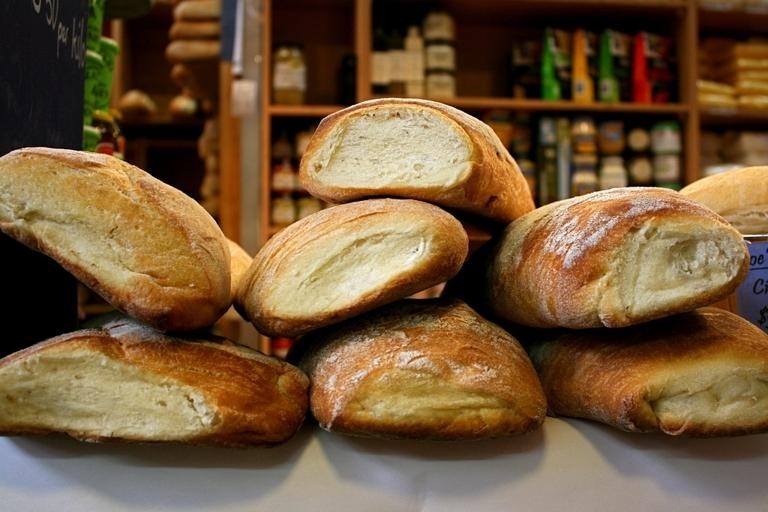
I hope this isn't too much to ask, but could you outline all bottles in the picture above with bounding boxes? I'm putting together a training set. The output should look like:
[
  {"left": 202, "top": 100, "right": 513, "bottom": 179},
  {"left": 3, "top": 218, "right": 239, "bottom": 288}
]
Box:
[{"left": 403, "top": 28, "right": 425, "bottom": 98}]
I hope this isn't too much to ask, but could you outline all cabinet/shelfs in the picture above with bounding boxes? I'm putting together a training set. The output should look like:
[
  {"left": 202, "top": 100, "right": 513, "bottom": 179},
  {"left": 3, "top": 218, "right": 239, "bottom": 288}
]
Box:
[{"left": 259, "top": 2, "right": 768, "bottom": 358}]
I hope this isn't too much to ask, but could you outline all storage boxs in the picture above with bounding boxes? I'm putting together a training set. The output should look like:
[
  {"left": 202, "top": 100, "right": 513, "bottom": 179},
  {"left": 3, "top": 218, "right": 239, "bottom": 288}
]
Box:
[{"left": 708, "top": 233, "right": 768, "bottom": 332}]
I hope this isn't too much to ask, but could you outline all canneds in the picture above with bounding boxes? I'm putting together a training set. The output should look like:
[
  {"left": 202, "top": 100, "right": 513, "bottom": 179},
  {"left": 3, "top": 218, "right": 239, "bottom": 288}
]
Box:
[
  {"left": 273, "top": 44, "right": 307, "bottom": 106},
  {"left": 425, "top": 10, "right": 457, "bottom": 99},
  {"left": 572, "top": 118, "right": 681, "bottom": 192},
  {"left": 90, "top": 108, "right": 126, "bottom": 158},
  {"left": 271, "top": 127, "right": 333, "bottom": 224}
]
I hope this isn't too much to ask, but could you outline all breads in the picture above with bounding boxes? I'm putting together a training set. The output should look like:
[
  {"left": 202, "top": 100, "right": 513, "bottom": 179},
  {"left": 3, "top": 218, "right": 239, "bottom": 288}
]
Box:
[
  {"left": 676, "top": 163, "right": 764, "bottom": 235},
  {"left": 1, "top": 318, "right": 308, "bottom": 447},
  {"left": 168, "top": 1, "right": 221, "bottom": 217},
  {"left": 297, "top": 98, "right": 538, "bottom": 240},
  {"left": 234, "top": 196, "right": 471, "bottom": 337},
  {"left": 120, "top": 89, "right": 155, "bottom": 112},
  {"left": 0, "top": 145, "right": 253, "bottom": 335},
  {"left": 522, "top": 304, "right": 768, "bottom": 438},
  {"left": 287, "top": 299, "right": 546, "bottom": 439},
  {"left": 443, "top": 185, "right": 750, "bottom": 332}
]
